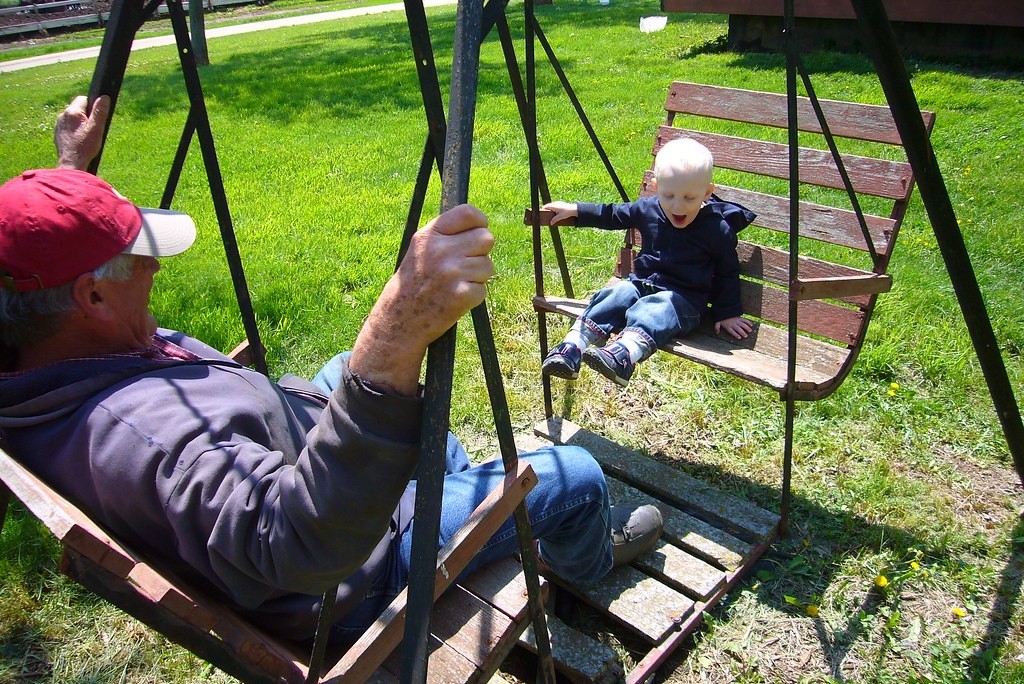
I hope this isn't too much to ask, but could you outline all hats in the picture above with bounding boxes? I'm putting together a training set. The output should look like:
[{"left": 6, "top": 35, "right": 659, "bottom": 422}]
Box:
[{"left": 0, "top": 168, "right": 196, "bottom": 292}]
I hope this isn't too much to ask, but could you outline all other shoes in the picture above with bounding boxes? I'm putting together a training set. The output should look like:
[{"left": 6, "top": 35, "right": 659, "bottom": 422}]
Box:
[{"left": 532, "top": 504, "right": 663, "bottom": 574}]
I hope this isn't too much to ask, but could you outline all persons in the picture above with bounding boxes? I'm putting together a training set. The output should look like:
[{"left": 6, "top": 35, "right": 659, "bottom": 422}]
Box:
[
  {"left": 0, "top": 94, "right": 663, "bottom": 667},
  {"left": 541, "top": 137, "right": 757, "bottom": 388}
]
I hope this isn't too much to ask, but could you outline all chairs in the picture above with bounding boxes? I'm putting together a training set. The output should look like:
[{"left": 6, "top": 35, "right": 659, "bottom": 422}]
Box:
[
  {"left": 523, "top": 81, "right": 936, "bottom": 401},
  {"left": 0, "top": 417, "right": 549, "bottom": 684}
]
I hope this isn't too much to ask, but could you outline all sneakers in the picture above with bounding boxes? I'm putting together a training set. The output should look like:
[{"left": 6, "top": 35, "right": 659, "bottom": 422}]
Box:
[
  {"left": 542, "top": 342, "right": 582, "bottom": 380},
  {"left": 582, "top": 343, "right": 634, "bottom": 387}
]
